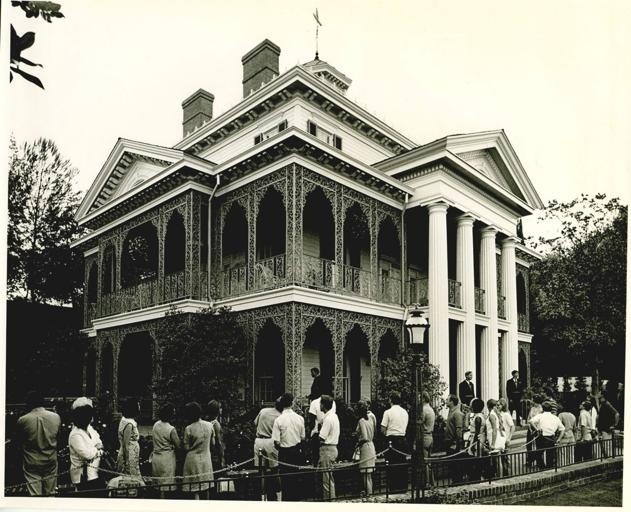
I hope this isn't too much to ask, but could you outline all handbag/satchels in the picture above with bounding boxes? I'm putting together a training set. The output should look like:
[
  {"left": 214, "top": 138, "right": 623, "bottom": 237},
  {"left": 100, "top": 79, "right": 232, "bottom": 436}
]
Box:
[
  {"left": 352, "top": 442, "right": 361, "bottom": 461},
  {"left": 216, "top": 465, "right": 235, "bottom": 493}
]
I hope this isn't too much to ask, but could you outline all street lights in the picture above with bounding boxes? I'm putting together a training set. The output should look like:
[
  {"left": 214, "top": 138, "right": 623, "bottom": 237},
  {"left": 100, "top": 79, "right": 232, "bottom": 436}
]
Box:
[{"left": 402, "top": 305, "right": 429, "bottom": 491}]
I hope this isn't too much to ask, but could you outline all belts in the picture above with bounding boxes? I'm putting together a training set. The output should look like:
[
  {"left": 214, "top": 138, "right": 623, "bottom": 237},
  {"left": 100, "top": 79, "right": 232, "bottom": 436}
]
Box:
[
  {"left": 321, "top": 443, "right": 338, "bottom": 447},
  {"left": 255, "top": 435, "right": 272, "bottom": 439}
]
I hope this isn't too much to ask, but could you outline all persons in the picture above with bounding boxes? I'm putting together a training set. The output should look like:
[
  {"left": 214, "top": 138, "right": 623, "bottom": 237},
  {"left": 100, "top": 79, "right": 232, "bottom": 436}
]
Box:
[
  {"left": 468, "top": 398, "right": 487, "bottom": 484},
  {"left": 352, "top": 401, "right": 376, "bottom": 497},
  {"left": 486, "top": 399, "right": 505, "bottom": 478},
  {"left": 317, "top": 395, "right": 340, "bottom": 501},
  {"left": 205, "top": 399, "right": 224, "bottom": 478},
  {"left": 577, "top": 400, "right": 593, "bottom": 461},
  {"left": 597, "top": 390, "right": 620, "bottom": 457},
  {"left": 272, "top": 398, "right": 305, "bottom": 501},
  {"left": 444, "top": 393, "right": 463, "bottom": 487},
  {"left": 418, "top": 392, "right": 435, "bottom": 489},
  {"left": 497, "top": 398, "right": 515, "bottom": 476},
  {"left": 507, "top": 370, "right": 522, "bottom": 426},
  {"left": 542, "top": 387, "right": 557, "bottom": 414},
  {"left": 181, "top": 402, "right": 215, "bottom": 499},
  {"left": 306, "top": 367, "right": 323, "bottom": 399},
  {"left": 309, "top": 385, "right": 337, "bottom": 467},
  {"left": 380, "top": 392, "right": 409, "bottom": 493},
  {"left": 152, "top": 404, "right": 180, "bottom": 499},
  {"left": 587, "top": 396, "right": 598, "bottom": 459},
  {"left": 459, "top": 371, "right": 474, "bottom": 406},
  {"left": 69, "top": 398, "right": 103, "bottom": 490},
  {"left": 253, "top": 401, "right": 282, "bottom": 469},
  {"left": 524, "top": 393, "right": 542, "bottom": 466},
  {"left": 558, "top": 401, "right": 576, "bottom": 467},
  {"left": 529, "top": 402, "right": 565, "bottom": 469},
  {"left": 16, "top": 392, "right": 61, "bottom": 497},
  {"left": 358, "top": 398, "right": 377, "bottom": 426},
  {"left": 118, "top": 398, "right": 146, "bottom": 486}
]
